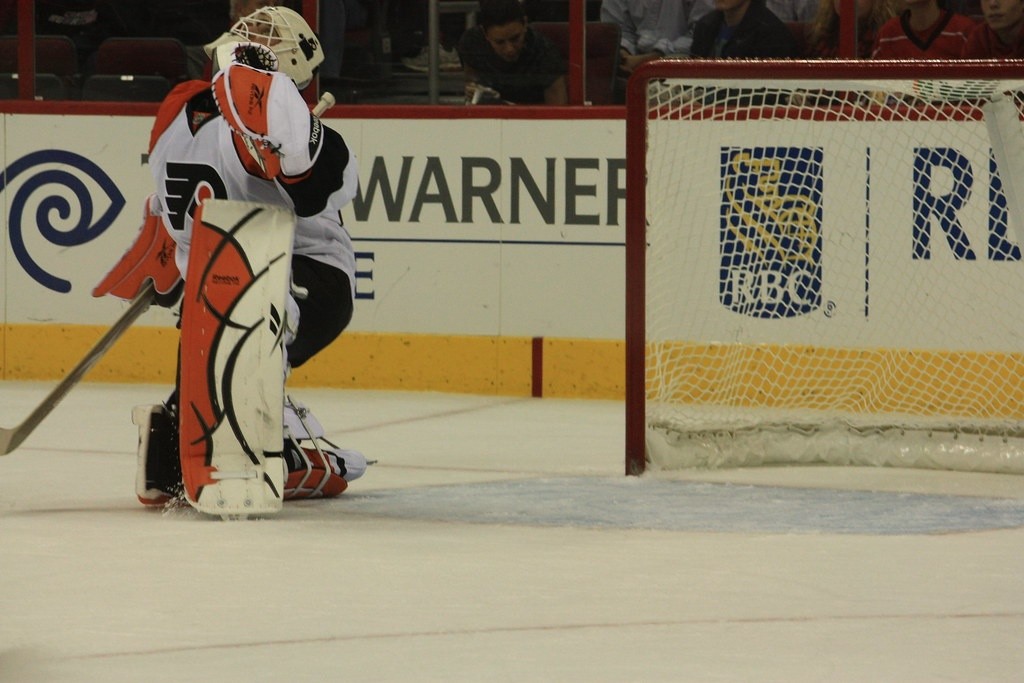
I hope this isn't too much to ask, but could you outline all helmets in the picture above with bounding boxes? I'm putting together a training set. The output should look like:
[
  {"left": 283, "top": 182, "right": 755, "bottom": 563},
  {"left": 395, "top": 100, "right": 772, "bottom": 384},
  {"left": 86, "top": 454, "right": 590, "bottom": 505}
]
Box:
[{"left": 204, "top": 6, "right": 324, "bottom": 91}]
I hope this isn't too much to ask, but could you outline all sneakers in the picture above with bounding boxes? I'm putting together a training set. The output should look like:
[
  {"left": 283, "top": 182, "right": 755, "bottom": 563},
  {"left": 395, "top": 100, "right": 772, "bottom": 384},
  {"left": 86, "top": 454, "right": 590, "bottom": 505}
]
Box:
[{"left": 402, "top": 44, "right": 462, "bottom": 72}]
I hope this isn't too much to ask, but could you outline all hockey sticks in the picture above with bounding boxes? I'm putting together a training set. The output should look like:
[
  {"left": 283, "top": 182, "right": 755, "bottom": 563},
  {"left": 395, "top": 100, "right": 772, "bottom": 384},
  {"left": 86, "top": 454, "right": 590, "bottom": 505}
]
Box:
[{"left": 1, "top": 88, "right": 335, "bottom": 455}]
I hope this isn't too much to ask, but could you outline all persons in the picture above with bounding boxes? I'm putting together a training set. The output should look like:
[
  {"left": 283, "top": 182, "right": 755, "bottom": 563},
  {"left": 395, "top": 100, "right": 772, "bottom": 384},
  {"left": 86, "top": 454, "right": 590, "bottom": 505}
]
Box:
[
  {"left": 403, "top": 0, "right": 1023, "bottom": 108},
  {"left": 95, "top": 6, "right": 367, "bottom": 523}
]
